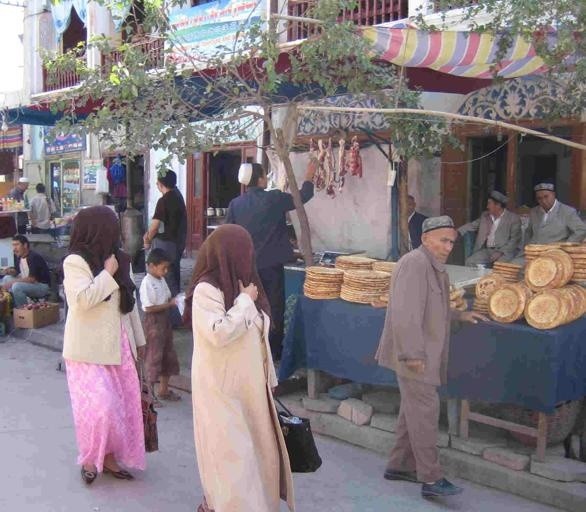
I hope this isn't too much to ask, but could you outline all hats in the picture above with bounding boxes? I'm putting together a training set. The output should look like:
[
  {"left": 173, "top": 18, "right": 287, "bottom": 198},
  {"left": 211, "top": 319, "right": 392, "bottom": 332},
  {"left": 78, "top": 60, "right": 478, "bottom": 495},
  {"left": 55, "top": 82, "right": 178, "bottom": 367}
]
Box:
[
  {"left": 19, "top": 177, "right": 28, "bottom": 182},
  {"left": 489, "top": 191, "right": 508, "bottom": 204},
  {"left": 158, "top": 170, "right": 177, "bottom": 188},
  {"left": 238, "top": 164, "right": 253, "bottom": 186},
  {"left": 421, "top": 215, "right": 455, "bottom": 232},
  {"left": 534, "top": 183, "right": 554, "bottom": 191}
]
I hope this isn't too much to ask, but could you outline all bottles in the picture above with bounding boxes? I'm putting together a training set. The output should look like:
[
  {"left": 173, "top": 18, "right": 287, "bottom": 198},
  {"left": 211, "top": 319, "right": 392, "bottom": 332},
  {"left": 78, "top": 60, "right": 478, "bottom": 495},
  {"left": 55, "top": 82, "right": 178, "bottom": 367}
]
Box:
[
  {"left": 0, "top": 197, "right": 23, "bottom": 212},
  {"left": 206, "top": 207, "right": 228, "bottom": 217}
]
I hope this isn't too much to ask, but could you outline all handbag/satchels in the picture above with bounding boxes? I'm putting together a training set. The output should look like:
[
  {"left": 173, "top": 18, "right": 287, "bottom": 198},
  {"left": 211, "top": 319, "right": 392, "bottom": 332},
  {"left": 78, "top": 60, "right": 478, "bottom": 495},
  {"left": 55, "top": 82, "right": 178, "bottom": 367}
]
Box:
[
  {"left": 136, "top": 358, "right": 158, "bottom": 451},
  {"left": 273, "top": 394, "right": 322, "bottom": 472}
]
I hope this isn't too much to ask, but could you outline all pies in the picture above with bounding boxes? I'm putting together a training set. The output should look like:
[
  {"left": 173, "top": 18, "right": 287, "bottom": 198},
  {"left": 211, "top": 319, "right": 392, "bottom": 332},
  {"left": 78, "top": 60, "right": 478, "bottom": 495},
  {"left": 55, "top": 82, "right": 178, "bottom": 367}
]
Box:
[
  {"left": 472, "top": 240, "right": 586, "bottom": 329},
  {"left": 304, "top": 256, "right": 396, "bottom": 307},
  {"left": 449, "top": 284, "right": 467, "bottom": 311}
]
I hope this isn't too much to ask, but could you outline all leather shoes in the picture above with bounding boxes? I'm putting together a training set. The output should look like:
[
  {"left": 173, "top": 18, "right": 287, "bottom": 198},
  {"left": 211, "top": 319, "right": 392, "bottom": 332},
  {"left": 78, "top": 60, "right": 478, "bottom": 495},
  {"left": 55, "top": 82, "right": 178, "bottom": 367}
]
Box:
[
  {"left": 81, "top": 462, "right": 98, "bottom": 484},
  {"left": 383, "top": 467, "right": 421, "bottom": 484},
  {"left": 103, "top": 463, "right": 133, "bottom": 481},
  {"left": 421, "top": 478, "right": 463, "bottom": 499}
]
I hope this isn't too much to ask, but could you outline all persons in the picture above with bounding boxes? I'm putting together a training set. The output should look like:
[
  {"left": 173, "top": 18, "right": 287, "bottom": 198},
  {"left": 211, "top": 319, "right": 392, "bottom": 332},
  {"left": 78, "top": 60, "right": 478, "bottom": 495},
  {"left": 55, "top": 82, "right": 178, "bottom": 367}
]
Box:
[
  {"left": 29, "top": 183, "right": 56, "bottom": 235},
  {"left": 98, "top": 192, "right": 119, "bottom": 221},
  {"left": 138, "top": 249, "right": 181, "bottom": 408},
  {"left": 376, "top": 214, "right": 486, "bottom": 497},
  {"left": 226, "top": 157, "right": 318, "bottom": 396},
  {"left": 183, "top": 224, "right": 296, "bottom": 512},
  {"left": 457, "top": 191, "right": 522, "bottom": 269},
  {"left": 0, "top": 235, "right": 50, "bottom": 308},
  {"left": 59, "top": 206, "right": 147, "bottom": 484},
  {"left": 143, "top": 168, "right": 187, "bottom": 329},
  {"left": 11, "top": 178, "right": 30, "bottom": 233},
  {"left": 512, "top": 181, "right": 586, "bottom": 267},
  {"left": 408, "top": 194, "right": 430, "bottom": 251}
]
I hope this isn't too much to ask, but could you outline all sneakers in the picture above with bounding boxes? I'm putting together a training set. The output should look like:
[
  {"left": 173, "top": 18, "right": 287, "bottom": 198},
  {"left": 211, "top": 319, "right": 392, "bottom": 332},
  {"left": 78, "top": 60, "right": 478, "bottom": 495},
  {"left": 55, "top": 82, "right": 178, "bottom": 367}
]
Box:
[
  {"left": 151, "top": 394, "right": 162, "bottom": 407},
  {"left": 158, "top": 392, "right": 181, "bottom": 402}
]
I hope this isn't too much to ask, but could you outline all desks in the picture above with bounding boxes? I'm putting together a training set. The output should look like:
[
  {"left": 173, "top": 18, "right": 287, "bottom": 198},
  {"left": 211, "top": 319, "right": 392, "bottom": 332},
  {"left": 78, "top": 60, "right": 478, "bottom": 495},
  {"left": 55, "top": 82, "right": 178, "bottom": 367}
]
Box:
[
  {"left": 453, "top": 294, "right": 585, "bottom": 463},
  {"left": 277, "top": 264, "right": 480, "bottom": 437}
]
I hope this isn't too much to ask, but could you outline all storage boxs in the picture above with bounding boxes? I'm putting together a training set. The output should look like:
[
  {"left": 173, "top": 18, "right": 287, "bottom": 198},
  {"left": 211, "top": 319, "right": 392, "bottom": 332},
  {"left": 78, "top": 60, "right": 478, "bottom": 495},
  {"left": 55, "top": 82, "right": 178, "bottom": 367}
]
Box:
[{"left": 11, "top": 300, "right": 60, "bottom": 330}]
